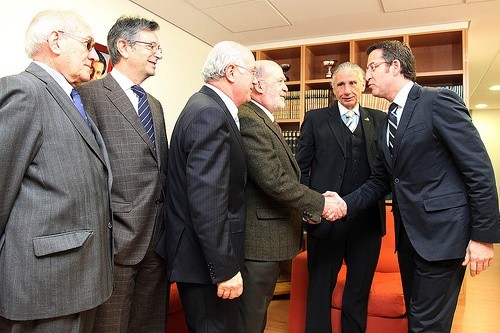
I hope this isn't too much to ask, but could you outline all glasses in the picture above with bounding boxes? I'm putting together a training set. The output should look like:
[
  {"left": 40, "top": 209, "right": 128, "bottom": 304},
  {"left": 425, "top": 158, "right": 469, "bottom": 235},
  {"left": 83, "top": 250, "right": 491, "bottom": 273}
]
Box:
[
  {"left": 233, "top": 64, "right": 259, "bottom": 78},
  {"left": 129, "top": 41, "right": 163, "bottom": 54},
  {"left": 46, "top": 30, "right": 94, "bottom": 52},
  {"left": 365, "top": 61, "right": 391, "bottom": 72}
]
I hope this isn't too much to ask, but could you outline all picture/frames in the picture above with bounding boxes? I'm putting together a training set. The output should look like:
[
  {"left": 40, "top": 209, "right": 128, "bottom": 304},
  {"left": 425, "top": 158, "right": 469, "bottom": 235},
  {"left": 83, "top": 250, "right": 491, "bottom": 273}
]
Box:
[{"left": 72, "top": 42, "right": 114, "bottom": 83}]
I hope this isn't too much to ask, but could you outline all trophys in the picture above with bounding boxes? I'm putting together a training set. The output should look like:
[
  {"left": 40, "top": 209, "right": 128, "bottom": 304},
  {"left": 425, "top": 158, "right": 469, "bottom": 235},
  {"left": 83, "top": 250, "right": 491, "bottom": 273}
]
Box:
[
  {"left": 322, "top": 60, "right": 335, "bottom": 80},
  {"left": 280, "top": 64, "right": 291, "bottom": 80}
]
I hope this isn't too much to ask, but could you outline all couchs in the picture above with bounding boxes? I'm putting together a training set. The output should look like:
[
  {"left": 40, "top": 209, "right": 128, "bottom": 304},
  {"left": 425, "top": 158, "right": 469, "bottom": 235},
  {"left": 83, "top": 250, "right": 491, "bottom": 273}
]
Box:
[
  {"left": 164, "top": 283, "right": 191, "bottom": 333},
  {"left": 291, "top": 205, "right": 410, "bottom": 333}
]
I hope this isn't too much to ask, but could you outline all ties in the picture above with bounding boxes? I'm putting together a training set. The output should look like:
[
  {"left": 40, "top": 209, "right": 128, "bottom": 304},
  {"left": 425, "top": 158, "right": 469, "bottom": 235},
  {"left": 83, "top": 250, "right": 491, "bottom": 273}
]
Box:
[
  {"left": 387, "top": 103, "right": 398, "bottom": 151},
  {"left": 70, "top": 89, "right": 90, "bottom": 127},
  {"left": 235, "top": 114, "right": 241, "bottom": 131},
  {"left": 344, "top": 110, "right": 357, "bottom": 133},
  {"left": 273, "top": 119, "right": 282, "bottom": 133},
  {"left": 130, "top": 85, "right": 156, "bottom": 146}
]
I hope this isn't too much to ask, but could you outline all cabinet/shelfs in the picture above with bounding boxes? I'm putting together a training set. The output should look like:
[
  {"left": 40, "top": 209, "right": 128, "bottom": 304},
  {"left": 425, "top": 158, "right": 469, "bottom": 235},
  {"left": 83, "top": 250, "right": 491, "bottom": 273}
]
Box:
[{"left": 214, "top": 23, "right": 470, "bottom": 171}]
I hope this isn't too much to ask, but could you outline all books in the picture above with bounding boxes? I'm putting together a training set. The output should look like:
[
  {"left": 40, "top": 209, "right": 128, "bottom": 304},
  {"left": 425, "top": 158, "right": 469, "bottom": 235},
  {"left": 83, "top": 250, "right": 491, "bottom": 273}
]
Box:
[{"left": 273, "top": 87, "right": 464, "bottom": 156}]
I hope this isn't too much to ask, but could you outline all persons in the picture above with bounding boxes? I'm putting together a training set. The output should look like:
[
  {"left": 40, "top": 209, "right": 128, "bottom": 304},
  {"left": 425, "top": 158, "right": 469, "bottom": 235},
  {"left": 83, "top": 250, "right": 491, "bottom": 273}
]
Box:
[
  {"left": 296, "top": 63, "right": 388, "bottom": 333},
  {"left": 0, "top": 9, "right": 112, "bottom": 333},
  {"left": 238, "top": 60, "right": 347, "bottom": 333},
  {"left": 322, "top": 41, "right": 500, "bottom": 333},
  {"left": 76, "top": 16, "right": 169, "bottom": 333},
  {"left": 90, "top": 47, "right": 107, "bottom": 81},
  {"left": 169, "top": 40, "right": 249, "bottom": 333}
]
